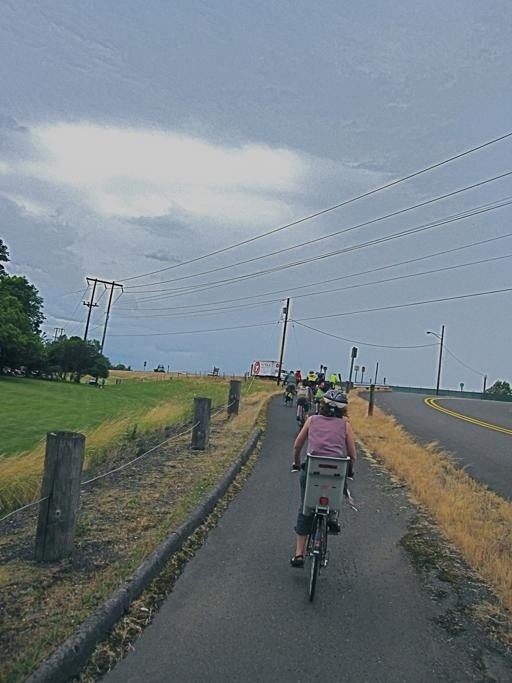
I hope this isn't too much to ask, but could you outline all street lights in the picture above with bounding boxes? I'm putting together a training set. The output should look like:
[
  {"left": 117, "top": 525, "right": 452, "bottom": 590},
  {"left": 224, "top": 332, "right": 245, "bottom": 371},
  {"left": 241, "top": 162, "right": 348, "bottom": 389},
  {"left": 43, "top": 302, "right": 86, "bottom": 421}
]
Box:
[{"left": 426, "top": 330, "right": 442, "bottom": 396}]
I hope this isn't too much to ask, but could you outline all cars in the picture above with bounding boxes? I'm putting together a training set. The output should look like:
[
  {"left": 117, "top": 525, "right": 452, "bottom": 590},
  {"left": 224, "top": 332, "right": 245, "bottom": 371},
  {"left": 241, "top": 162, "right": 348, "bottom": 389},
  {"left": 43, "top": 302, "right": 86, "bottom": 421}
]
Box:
[
  {"left": 2, "top": 363, "right": 101, "bottom": 387},
  {"left": 208, "top": 370, "right": 218, "bottom": 377}
]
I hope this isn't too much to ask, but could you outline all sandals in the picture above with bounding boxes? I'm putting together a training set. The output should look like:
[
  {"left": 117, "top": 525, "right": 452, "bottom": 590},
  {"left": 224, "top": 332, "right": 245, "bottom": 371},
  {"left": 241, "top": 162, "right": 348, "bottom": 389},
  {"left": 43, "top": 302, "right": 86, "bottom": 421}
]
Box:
[
  {"left": 327, "top": 516, "right": 340, "bottom": 532},
  {"left": 291, "top": 555, "right": 303, "bottom": 566}
]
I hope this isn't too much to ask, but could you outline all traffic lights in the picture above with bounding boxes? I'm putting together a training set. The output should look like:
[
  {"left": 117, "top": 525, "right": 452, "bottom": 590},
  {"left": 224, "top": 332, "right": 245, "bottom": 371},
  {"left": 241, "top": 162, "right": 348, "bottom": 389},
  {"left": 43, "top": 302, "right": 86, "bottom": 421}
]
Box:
[{"left": 352, "top": 348, "right": 358, "bottom": 358}]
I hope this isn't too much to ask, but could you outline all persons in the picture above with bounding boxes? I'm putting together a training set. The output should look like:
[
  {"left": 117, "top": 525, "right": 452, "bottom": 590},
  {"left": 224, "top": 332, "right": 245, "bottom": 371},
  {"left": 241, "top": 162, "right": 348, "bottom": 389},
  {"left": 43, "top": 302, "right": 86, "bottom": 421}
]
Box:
[
  {"left": 254, "top": 362, "right": 260, "bottom": 375},
  {"left": 289, "top": 390, "right": 357, "bottom": 569},
  {"left": 280, "top": 367, "right": 339, "bottom": 423}
]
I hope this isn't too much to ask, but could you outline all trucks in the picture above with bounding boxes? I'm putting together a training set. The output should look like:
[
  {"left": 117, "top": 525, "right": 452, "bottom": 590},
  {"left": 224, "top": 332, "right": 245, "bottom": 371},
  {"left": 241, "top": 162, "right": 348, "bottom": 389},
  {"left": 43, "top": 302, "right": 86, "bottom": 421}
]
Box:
[
  {"left": 251, "top": 358, "right": 288, "bottom": 381},
  {"left": 153, "top": 365, "right": 165, "bottom": 372}
]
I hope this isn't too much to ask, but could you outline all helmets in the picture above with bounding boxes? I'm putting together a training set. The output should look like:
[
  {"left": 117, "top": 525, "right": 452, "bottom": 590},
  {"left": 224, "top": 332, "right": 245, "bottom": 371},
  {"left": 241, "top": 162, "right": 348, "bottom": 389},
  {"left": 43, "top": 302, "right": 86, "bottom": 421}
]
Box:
[{"left": 323, "top": 390, "right": 348, "bottom": 408}]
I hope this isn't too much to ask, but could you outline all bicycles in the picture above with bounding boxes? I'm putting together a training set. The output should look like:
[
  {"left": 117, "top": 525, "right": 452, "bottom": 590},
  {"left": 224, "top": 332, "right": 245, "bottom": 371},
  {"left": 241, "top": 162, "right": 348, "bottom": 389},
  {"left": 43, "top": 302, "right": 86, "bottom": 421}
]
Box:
[
  {"left": 290, "top": 452, "right": 355, "bottom": 601},
  {"left": 282, "top": 375, "right": 339, "bottom": 434}
]
